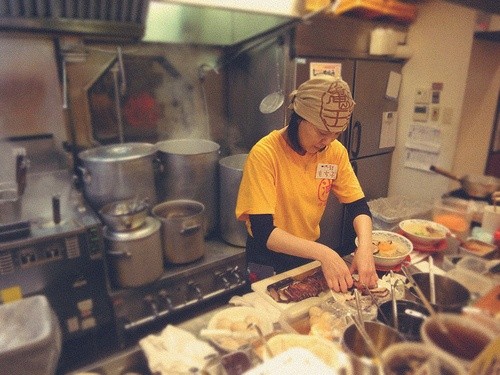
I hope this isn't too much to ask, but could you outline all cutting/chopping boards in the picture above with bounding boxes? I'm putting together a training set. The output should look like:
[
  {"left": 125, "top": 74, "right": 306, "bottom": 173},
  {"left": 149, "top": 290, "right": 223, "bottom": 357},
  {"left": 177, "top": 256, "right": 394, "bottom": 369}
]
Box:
[{"left": 253, "top": 259, "right": 332, "bottom": 319}]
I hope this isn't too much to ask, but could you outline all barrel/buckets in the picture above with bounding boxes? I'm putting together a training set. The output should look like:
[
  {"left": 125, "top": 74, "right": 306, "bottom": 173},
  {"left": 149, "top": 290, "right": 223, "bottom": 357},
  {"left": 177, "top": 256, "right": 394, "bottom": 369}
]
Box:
[
  {"left": 102, "top": 216, "right": 165, "bottom": 288},
  {"left": 218, "top": 154, "right": 248, "bottom": 248},
  {"left": 151, "top": 199, "right": 208, "bottom": 264},
  {"left": 76, "top": 142, "right": 158, "bottom": 216},
  {"left": 154, "top": 138, "right": 221, "bottom": 240}
]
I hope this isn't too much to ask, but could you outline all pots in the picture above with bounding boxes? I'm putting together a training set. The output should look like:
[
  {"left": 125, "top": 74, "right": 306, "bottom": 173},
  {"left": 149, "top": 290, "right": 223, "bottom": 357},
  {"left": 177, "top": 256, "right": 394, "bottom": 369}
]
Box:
[{"left": 430, "top": 166, "right": 500, "bottom": 199}]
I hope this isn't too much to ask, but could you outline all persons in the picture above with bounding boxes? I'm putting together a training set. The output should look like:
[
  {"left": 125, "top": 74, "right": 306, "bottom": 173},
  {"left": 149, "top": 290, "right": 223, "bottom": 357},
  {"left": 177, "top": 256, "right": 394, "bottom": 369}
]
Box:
[{"left": 235, "top": 74, "right": 379, "bottom": 292}]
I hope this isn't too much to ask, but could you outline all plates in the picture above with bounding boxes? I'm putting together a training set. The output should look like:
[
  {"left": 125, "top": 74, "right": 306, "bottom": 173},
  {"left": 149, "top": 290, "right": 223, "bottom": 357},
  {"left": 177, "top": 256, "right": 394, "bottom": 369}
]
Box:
[
  {"left": 375, "top": 255, "right": 411, "bottom": 271},
  {"left": 399, "top": 230, "right": 450, "bottom": 252}
]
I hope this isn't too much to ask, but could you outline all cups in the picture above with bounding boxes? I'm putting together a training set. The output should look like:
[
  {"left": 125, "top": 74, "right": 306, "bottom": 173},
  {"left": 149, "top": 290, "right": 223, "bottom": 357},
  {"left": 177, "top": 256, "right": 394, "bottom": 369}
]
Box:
[
  {"left": 421, "top": 315, "right": 500, "bottom": 371},
  {"left": 343, "top": 320, "right": 407, "bottom": 374},
  {"left": 405, "top": 272, "right": 469, "bottom": 314},
  {"left": 377, "top": 341, "right": 466, "bottom": 374},
  {"left": 481, "top": 206, "right": 500, "bottom": 234},
  {"left": 377, "top": 300, "right": 434, "bottom": 341}
]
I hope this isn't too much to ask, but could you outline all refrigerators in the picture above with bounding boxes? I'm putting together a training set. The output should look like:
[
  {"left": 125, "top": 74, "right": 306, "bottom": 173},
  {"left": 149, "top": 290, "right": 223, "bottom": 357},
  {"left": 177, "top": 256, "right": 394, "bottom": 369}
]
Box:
[{"left": 227, "top": 55, "right": 400, "bottom": 259}]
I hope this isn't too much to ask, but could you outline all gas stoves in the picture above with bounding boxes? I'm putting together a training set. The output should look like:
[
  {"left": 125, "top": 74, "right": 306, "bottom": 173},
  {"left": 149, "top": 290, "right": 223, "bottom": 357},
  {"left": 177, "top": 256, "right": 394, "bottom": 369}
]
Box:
[{"left": 440, "top": 187, "right": 500, "bottom": 224}]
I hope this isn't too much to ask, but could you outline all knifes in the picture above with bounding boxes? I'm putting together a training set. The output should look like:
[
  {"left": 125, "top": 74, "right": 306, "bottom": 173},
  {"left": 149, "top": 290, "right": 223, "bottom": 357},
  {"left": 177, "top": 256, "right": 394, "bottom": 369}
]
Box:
[{"left": 267, "top": 265, "right": 321, "bottom": 291}]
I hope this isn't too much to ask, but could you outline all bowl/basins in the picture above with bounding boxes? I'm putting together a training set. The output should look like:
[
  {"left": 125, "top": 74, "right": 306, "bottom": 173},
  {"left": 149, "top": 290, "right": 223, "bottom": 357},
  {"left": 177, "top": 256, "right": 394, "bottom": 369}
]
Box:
[
  {"left": 276, "top": 297, "right": 356, "bottom": 344},
  {"left": 98, "top": 199, "right": 149, "bottom": 231},
  {"left": 457, "top": 236, "right": 498, "bottom": 259},
  {"left": 208, "top": 306, "right": 274, "bottom": 351},
  {"left": 399, "top": 220, "right": 451, "bottom": 245},
  {"left": 433, "top": 197, "right": 475, "bottom": 232},
  {"left": 354, "top": 231, "right": 411, "bottom": 266},
  {"left": 443, "top": 254, "right": 500, "bottom": 317},
  {"left": 262, "top": 335, "right": 342, "bottom": 374}
]
